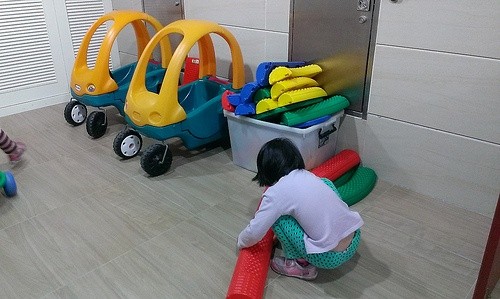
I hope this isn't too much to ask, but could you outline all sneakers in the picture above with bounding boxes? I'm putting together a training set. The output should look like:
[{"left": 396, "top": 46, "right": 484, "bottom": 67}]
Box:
[{"left": 270, "top": 256, "right": 318, "bottom": 280}]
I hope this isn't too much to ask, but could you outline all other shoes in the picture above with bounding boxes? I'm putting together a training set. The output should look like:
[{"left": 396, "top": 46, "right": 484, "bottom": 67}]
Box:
[
  {"left": 10, "top": 141, "right": 27, "bottom": 161},
  {"left": 4, "top": 171, "right": 17, "bottom": 197}
]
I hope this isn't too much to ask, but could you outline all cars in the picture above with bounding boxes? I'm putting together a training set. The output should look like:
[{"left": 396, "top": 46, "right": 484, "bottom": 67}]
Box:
[
  {"left": 64, "top": 10, "right": 174, "bottom": 137},
  {"left": 112, "top": 18, "right": 249, "bottom": 177}
]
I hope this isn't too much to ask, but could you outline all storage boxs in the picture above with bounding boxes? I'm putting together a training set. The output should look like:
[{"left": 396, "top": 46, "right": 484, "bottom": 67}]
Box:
[{"left": 223, "top": 108, "right": 345, "bottom": 174}]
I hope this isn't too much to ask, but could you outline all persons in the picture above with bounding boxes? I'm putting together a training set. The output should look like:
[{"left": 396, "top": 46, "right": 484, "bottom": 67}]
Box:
[
  {"left": 0, "top": 128, "right": 27, "bottom": 160},
  {"left": 236, "top": 137, "right": 365, "bottom": 280}
]
